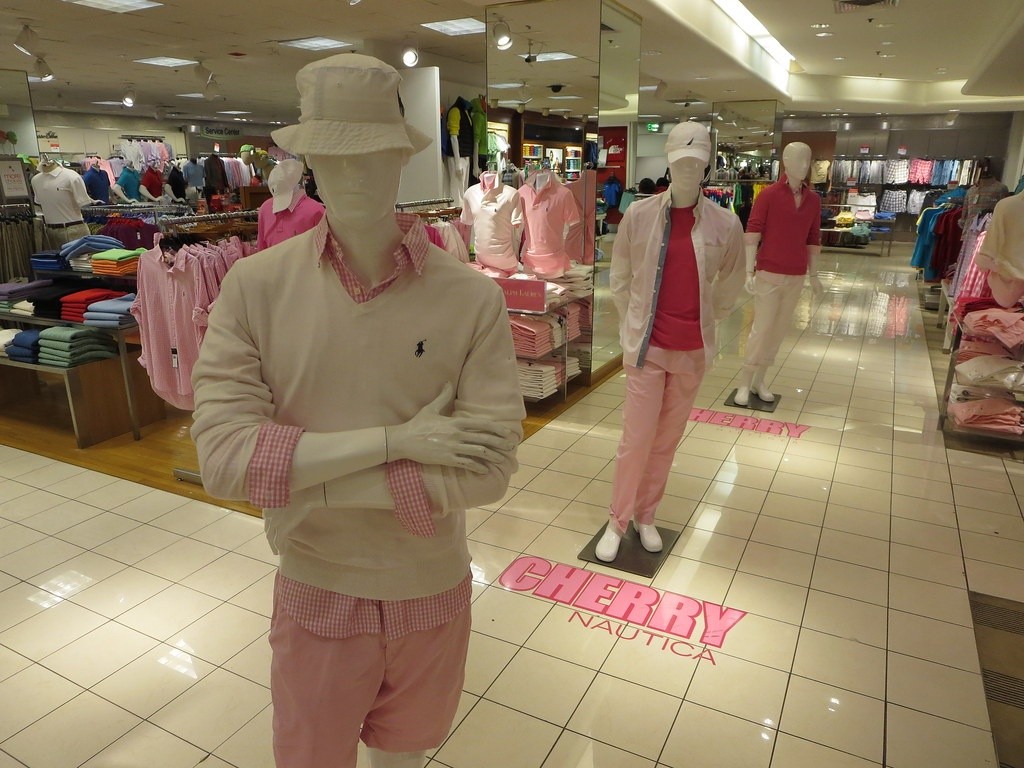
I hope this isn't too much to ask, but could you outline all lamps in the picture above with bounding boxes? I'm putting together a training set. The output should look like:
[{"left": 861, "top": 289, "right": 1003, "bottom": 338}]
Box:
[
  {"left": 542, "top": 108, "right": 549, "bottom": 116},
  {"left": 123, "top": 90, "right": 137, "bottom": 107},
  {"left": 494, "top": 20, "right": 514, "bottom": 50},
  {"left": 35, "top": 59, "right": 53, "bottom": 82},
  {"left": 489, "top": 99, "right": 498, "bottom": 109},
  {"left": 402, "top": 46, "right": 419, "bottom": 67},
  {"left": 563, "top": 111, "right": 571, "bottom": 119},
  {"left": 583, "top": 114, "right": 589, "bottom": 123},
  {"left": 13, "top": 25, "right": 38, "bottom": 56},
  {"left": 517, "top": 104, "right": 525, "bottom": 113}
]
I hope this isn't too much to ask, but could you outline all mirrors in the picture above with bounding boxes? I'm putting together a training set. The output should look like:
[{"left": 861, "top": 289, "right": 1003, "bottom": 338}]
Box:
[
  {"left": 712, "top": 99, "right": 786, "bottom": 183},
  {"left": 485, "top": 0, "right": 641, "bottom": 389}
]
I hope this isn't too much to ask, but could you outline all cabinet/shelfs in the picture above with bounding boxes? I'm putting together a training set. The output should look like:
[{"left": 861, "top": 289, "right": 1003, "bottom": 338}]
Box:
[
  {"left": 821, "top": 216, "right": 895, "bottom": 257},
  {"left": 523, "top": 142, "right": 544, "bottom": 170},
  {"left": 565, "top": 145, "right": 582, "bottom": 180}
]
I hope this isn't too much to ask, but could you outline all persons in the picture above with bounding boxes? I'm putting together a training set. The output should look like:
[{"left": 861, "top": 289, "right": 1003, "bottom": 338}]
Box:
[
  {"left": 164, "top": 165, "right": 187, "bottom": 205},
  {"left": 82, "top": 164, "right": 110, "bottom": 224},
  {"left": 183, "top": 158, "right": 203, "bottom": 199},
  {"left": 30, "top": 158, "right": 91, "bottom": 250},
  {"left": 191, "top": 54, "right": 527, "bottom": 768},
  {"left": 204, "top": 152, "right": 228, "bottom": 203},
  {"left": 114, "top": 167, "right": 141, "bottom": 203},
  {"left": 596, "top": 121, "right": 747, "bottom": 561},
  {"left": 470, "top": 98, "right": 488, "bottom": 186},
  {"left": 564, "top": 170, "right": 596, "bottom": 266},
  {"left": 461, "top": 171, "right": 523, "bottom": 269},
  {"left": 735, "top": 142, "right": 823, "bottom": 405},
  {"left": 257, "top": 158, "right": 325, "bottom": 252},
  {"left": 139, "top": 166, "right": 166, "bottom": 202},
  {"left": 446, "top": 96, "right": 474, "bottom": 207},
  {"left": 518, "top": 173, "right": 580, "bottom": 277}
]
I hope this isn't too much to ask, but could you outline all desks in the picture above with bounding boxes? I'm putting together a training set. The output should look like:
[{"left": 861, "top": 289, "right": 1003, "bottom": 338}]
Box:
[
  {"left": 935, "top": 279, "right": 996, "bottom": 416},
  {"left": 0, "top": 311, "right": 144, "bottom": 441}
]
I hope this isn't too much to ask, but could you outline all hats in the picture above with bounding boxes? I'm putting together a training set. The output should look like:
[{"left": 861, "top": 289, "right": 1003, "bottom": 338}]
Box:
[
  {"left": 269, "top": 158, "right": 304, "bottom": 214},
  {"left": 271, "top": 54, "right": 433, "bottom": 154},
  {"left": 666, "top": 122, "right": 711, "bottom": 162},
  {"left": 239, "top": 144, "right": 254, "bottom": 154}
]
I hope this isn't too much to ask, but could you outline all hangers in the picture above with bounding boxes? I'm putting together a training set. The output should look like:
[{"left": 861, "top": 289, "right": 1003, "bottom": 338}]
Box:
[
  {"left": 942, "top": 197, "right": 995, "bottom": 217},
  {"left": 0, "top": 204, "right": 35, "bottom": 224},
  {"left": 107, "top": 137, "right": 241, "bottom": 165},
  {"left": 701, "top": 181, "right": 733, "bottom": 204},
  {"left": 81, "top": 203, "right": 260, "bottom": 252}
]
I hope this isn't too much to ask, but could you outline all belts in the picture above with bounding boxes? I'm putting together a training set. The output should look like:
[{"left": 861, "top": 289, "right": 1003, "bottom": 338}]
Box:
[{"left": 46, "top": 220, "right": 86, "bottom": 229}]
